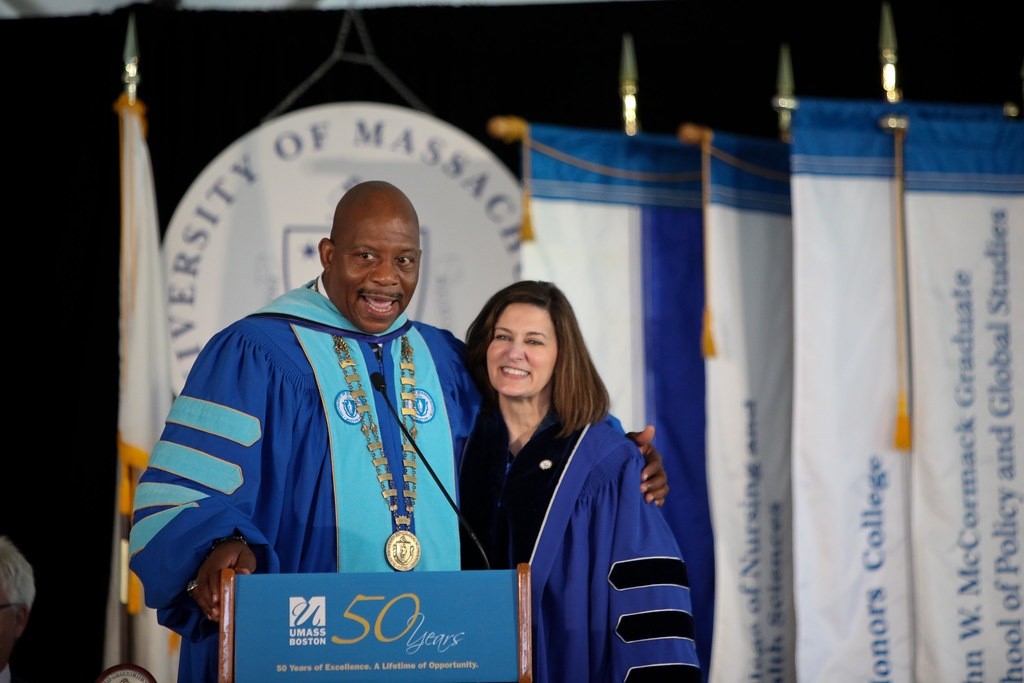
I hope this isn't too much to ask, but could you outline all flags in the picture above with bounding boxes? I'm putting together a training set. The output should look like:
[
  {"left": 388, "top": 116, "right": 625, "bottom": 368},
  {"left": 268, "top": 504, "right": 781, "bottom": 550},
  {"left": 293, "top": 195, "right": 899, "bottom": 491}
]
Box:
[{"left": 115, "top": 92, "right": 181, "bottom": 654}]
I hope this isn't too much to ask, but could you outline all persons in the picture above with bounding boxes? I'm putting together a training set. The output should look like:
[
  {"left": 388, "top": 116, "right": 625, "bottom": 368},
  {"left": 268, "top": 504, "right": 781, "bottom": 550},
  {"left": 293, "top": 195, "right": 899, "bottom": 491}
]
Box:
[
  {"left": 0, "top": 538, "right": 37, "bottom": 683},
  {"left": 126, "top": 180, "right": 702, "bottom": 683}
]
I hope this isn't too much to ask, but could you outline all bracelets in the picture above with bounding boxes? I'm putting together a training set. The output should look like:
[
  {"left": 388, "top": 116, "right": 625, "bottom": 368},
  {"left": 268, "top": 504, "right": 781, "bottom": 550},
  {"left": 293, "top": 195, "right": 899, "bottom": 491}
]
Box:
[{"left": 186, "top": 535, "right": 248, "bottom": 598}]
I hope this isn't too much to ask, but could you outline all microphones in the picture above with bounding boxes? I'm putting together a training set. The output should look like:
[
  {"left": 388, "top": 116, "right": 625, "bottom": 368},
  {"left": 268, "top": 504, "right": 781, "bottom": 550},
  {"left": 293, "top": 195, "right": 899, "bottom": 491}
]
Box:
[{"left": 370, "top": 371, "right": 491, "bottom": 570}]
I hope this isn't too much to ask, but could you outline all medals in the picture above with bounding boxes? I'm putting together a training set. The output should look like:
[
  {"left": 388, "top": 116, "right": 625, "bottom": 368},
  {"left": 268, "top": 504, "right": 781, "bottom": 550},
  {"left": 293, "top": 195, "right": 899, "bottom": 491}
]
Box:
[{"left": 333, "top": 334, "right": 421, "bottom": 571}]
